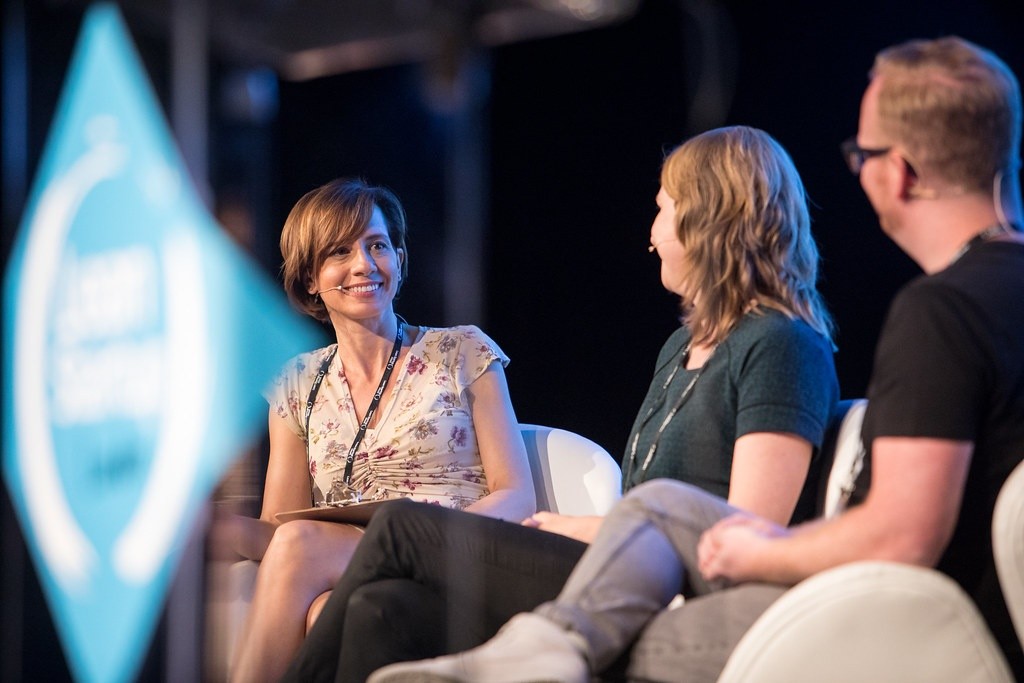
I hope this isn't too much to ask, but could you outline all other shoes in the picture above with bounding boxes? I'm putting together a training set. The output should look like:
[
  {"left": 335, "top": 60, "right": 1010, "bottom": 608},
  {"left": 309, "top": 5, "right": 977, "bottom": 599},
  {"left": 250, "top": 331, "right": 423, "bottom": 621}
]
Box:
[{"left": 367, "top": 612, "right": 592, "bottom": 683}]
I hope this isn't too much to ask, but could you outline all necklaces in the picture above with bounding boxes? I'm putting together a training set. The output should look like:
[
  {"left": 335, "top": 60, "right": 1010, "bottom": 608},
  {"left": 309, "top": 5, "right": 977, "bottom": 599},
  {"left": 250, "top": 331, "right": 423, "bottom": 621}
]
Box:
[
  {"left": 303, "top": 316, "right": 404, "bottom": 508},
  {"left": 624, "top": 330, "right": 720, "bottom": 494}
]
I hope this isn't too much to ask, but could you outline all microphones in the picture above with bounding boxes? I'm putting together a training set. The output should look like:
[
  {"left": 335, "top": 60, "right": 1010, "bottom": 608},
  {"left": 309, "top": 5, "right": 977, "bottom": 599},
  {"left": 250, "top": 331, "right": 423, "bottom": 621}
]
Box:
[
  {"left": 649, "top": 238, "right": 677, "bottom": 252},
  {"left": 319, "top": 286, "right": 342, "bottom": 293}
]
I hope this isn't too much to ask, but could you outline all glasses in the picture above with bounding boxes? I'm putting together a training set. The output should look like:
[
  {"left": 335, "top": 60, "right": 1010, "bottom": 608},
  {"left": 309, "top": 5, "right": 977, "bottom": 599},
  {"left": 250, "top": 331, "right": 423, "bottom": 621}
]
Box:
[{"left": 845, "top": 143, "right": 916, "bottom": 179}]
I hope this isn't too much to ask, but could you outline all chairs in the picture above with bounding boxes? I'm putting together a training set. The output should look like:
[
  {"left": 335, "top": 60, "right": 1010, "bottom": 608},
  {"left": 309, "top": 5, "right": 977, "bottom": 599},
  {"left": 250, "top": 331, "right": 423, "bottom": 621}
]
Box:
[{"left": 520, "top": 424, "right": 623, "bottom": 514}]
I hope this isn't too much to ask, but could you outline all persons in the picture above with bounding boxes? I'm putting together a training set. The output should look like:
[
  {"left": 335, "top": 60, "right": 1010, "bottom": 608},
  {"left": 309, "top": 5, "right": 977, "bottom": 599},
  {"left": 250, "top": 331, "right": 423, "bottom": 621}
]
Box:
[
  {"left": 364, "top": 37, "right": 1024, "bottom": 682},
  {"left": 229, "top": 182, "right": 538, "bottom": 683},
  {"left": 277, "top": 126, "right": 842, "bottom": 683}
]
